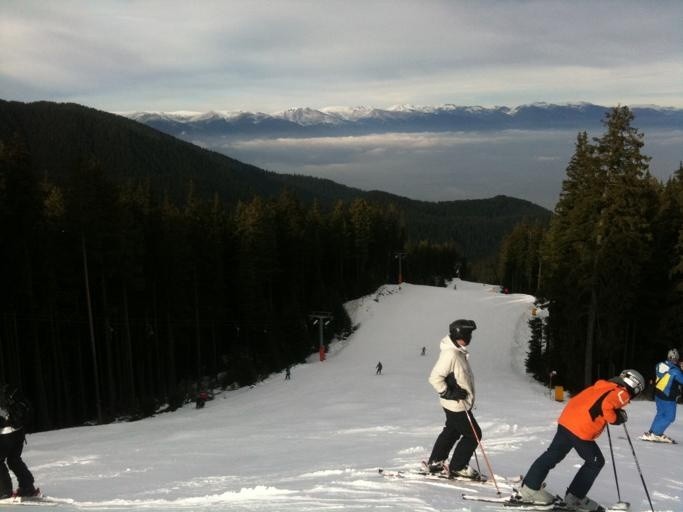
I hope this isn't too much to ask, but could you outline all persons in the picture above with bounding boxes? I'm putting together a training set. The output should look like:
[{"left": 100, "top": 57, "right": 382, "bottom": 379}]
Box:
[
  {"left": 510, "top": 367, "right": 646, "bottom": 512},
  {"left": 195, "top": 389, "right": 208, "bottom": 408},
  {"left": 376, "top": 360, "right": 383, "bottom": 376},
  {"left": 0, "top": 372, "right": 42, "bottom": 502},
  {"left": 284, "top": 365, "right": 291, "bottom": 380},
  {"left": 641, "top": 346, "right": 683, "bottom": 444}
]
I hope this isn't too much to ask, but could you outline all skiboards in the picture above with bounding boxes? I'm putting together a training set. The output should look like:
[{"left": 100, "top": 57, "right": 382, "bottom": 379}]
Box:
[
  {"left": 461, "top": 492, "right": 630, "bottom": 511},
  {"left": 378, "top": 462, "right": 524, "bottom": 491}
]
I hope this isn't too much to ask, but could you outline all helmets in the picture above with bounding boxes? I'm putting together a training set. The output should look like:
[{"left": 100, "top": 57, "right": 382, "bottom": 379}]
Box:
[
  {"left": 667, "top": 348, "right": 680, "bottom": 365},
  {"left": 618, "top": 369, "right": 645, "bottom": 397},
  {"left": 450, "top": 320, "right": 476, "bottom": 347}
]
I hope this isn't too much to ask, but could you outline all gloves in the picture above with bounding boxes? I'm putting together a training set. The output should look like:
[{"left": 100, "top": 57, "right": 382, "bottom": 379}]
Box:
[
  {"left": 609, "top": 409, "right": 628, "bottom": 425},
  {"left": 440, "top": 387, "right": 467, "bottom": 400}
]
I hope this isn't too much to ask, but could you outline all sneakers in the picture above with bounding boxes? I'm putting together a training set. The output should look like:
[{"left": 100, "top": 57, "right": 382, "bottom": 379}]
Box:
[
  {"left": 642, "top": 431, "right": 672, "bottom": 442},
  {"left": 518, "top": 483, "right": 554, "bottom": 505},
  {"left": 564, "top": 492, "right": 606, "bottom": 511},
  {"left": 428, "top": 461, "right": 488, "bottom": 482}
]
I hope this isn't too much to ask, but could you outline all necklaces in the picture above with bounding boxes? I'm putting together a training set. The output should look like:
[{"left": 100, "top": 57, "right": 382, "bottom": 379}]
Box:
[{"left": 417, "top": 318, "right": 489, "bottom": 482}]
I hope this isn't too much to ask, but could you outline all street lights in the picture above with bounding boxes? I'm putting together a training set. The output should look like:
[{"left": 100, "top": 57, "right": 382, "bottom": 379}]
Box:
[{"left": 547, "top": 369, "right": 556, "bottom": 400}]
